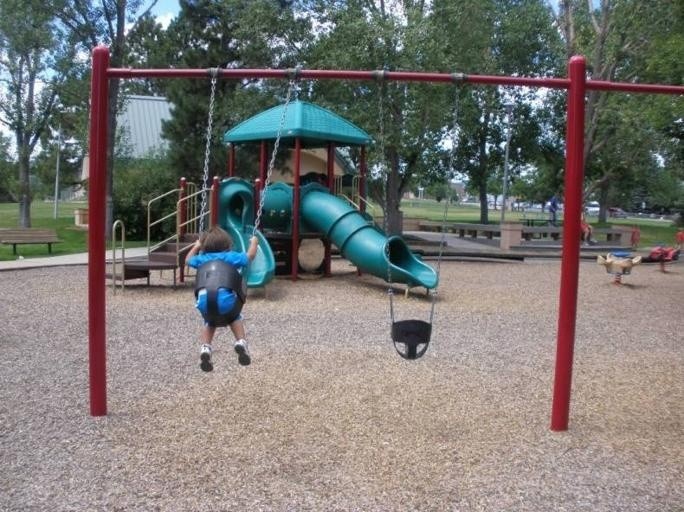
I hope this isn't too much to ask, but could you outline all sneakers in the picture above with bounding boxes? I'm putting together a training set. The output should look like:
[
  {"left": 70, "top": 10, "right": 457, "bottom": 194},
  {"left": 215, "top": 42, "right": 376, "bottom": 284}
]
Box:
[
  {"left": 234, "top": 339, "right": 251, "bottom": 365},
  {"left": 200, "top": 344, "right": 213, "bottom": 372}
]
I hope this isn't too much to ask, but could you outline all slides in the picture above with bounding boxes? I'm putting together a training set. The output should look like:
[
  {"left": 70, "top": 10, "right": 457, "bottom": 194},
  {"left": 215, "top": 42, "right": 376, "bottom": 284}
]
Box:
[
  {"left": 218, "top": 176, "right": 275, "bottom": 288},
  {"left": 302, "top": 191, "right": 438, "bottom": 288}
]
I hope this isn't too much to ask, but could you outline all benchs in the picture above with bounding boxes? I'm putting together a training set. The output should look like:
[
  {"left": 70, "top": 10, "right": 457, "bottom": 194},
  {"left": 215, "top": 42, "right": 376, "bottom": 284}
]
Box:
[{"left": 0, "top": 227, "right": 67, "bottom": 255}]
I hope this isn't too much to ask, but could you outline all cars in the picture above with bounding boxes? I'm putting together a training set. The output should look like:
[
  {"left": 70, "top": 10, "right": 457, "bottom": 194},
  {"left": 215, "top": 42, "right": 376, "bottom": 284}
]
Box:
[{"left": 606, "top": 206, "right": 628, "bottom": 219}]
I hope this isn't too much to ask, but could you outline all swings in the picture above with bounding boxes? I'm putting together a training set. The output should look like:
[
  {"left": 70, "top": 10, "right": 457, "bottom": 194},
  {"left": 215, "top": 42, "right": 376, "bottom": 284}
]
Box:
[
  {"left": 377, "top": 79, "right": 460, "bottom": 359},
  {"left": 195, "top": 67, "right": 301, "bottom": 327}
]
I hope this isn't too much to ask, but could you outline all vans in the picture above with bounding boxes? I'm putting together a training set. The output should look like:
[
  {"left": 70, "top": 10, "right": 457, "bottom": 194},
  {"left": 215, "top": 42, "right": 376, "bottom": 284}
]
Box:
[{"left": 584, "top": 200, "right": 599, "bottom": 217}]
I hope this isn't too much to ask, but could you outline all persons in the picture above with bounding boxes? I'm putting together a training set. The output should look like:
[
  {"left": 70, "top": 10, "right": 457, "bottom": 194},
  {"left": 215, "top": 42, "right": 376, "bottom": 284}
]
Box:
[
  {"left": 548, "top": 196, "right": 562, "bottom": 226},
  {"left": 581, "top": 200, "right": 598, "bottom": 247},
  {"left": 184, "top": 225, "right": 259, "bottom": 372}
]
